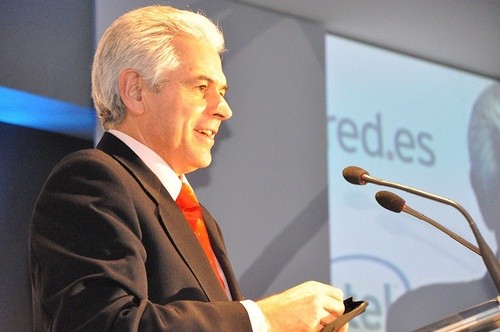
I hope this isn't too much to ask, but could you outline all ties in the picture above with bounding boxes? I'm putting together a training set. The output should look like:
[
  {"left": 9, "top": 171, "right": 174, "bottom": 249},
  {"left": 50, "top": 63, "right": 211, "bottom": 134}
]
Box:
[{"left": 175, "top": 182, "right": 226, "bottom": 296}]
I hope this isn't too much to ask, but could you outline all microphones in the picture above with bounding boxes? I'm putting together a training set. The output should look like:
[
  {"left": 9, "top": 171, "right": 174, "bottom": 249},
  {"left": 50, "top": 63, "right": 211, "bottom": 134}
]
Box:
[
  {"left": 343, "top": 166, "right": 500, "bottom": 292},
  {"left": 375, "top": 191, "right": 482, "bottom": 257}
]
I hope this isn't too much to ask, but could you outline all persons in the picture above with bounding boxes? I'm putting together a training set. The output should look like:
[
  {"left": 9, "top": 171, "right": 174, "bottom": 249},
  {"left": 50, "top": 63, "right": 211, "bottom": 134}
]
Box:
[{"left": 29, "top": 5, "right": 346, "bottom": 332}]
